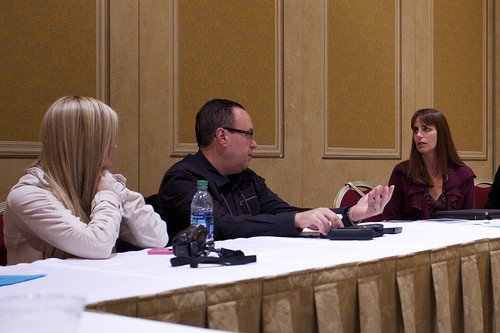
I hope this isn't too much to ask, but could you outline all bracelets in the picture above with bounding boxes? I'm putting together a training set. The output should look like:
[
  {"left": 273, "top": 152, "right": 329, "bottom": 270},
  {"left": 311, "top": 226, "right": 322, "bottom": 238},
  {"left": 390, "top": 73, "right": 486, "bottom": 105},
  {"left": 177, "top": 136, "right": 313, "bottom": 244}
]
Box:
[{"left": 346, "top": 206, "right": 362, "bottom": 226}]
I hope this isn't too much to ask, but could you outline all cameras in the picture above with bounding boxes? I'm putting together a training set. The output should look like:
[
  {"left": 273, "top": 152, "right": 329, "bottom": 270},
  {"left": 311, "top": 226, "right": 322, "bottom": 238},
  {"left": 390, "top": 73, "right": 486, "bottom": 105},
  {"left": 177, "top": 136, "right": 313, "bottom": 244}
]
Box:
[{"left": 173, "top": 224, "right": 209, "bottom": 258}]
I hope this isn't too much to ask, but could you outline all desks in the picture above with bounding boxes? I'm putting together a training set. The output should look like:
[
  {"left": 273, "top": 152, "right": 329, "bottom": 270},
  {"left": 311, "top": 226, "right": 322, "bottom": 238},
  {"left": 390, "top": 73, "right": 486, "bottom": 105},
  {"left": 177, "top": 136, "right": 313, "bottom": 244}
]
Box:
[{"left": 0, "top": 218, "right": 500, "bottom": 333}]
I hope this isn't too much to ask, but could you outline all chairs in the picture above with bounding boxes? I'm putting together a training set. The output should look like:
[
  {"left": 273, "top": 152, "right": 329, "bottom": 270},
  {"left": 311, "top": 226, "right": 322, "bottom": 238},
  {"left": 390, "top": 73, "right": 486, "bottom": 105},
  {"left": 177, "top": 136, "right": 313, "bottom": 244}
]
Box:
[
  {"left": 474, "top": 179, "right": 494, "bottom": 209},
  {"left": 334, "top": 181, "right": 383, "bottom": 223}
]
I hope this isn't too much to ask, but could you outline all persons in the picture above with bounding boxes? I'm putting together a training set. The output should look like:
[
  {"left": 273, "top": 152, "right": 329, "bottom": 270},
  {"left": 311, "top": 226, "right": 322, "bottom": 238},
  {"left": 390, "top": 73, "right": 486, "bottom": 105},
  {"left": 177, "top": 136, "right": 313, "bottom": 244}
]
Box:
[
  {"left": 378, "top": 108, "right": 477, "bottom": 222},
  {"left": 155, "top": 97, "right": 395, "bottom": 248},
  {"left": 4, "top": 95, "right": 170, "bottom": 267},
  {"left": 482, "top": 165, "right": 500, "bottom": 220}
]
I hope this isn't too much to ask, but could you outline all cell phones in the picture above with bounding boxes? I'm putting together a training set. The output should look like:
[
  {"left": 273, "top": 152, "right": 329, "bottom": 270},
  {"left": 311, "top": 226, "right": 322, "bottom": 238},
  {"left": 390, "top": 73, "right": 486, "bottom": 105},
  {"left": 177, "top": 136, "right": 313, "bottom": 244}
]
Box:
[{"left": 148, "top": 248, "right": 174, "bottom": 254}]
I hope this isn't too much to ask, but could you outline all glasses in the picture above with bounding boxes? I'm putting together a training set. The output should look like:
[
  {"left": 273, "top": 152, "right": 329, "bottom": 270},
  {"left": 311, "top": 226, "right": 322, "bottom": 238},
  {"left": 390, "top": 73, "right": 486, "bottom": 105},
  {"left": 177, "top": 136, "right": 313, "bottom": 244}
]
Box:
[{"left": 215, "top": 126, "right": 254, "bottom": 140}]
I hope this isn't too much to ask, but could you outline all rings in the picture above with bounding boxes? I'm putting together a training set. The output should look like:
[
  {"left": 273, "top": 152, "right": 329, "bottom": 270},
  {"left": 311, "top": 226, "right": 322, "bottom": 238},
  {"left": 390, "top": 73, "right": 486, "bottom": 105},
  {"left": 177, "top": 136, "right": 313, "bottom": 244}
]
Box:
[{"left": 373, "top": 204, "right": 377, "bottom": 210}]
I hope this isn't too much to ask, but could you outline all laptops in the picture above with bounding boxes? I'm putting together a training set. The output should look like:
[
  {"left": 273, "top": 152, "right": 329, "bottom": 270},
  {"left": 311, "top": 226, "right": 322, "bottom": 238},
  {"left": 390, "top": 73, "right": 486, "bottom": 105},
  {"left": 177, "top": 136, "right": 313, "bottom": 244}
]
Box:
[{"left": 435, "top": 209, "right": 500, "bottom": 220}]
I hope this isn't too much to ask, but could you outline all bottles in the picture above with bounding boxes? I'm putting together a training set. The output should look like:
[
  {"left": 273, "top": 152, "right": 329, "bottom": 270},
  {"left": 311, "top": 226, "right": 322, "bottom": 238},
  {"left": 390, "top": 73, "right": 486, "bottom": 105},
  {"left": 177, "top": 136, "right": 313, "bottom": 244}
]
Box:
[{"left": 190, "top": 180, "right": 213, "bottom": 241}]
